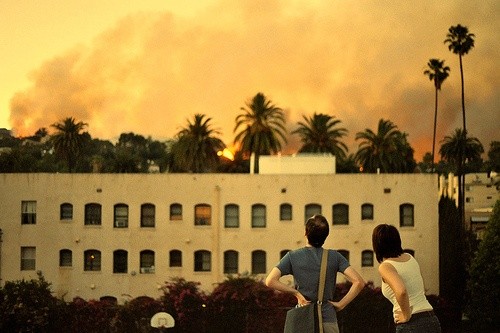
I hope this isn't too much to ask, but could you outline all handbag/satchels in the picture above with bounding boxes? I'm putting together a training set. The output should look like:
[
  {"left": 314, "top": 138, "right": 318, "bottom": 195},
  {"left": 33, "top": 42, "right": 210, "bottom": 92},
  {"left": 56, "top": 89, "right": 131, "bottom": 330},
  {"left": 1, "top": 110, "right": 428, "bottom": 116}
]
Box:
[{"left": 283, "top": 300, "right": 324, "bottom": 333}]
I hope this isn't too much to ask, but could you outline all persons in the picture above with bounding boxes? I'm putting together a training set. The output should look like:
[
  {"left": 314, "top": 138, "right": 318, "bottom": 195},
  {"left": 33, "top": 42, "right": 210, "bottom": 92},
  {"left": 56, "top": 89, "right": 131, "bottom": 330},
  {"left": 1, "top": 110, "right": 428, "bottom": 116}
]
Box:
[
  {"left": 265, "top": 214, "right": 365, "bottom": 333},
  {"left": 372, "top": 224, "right": 442, "bottom": 333}
]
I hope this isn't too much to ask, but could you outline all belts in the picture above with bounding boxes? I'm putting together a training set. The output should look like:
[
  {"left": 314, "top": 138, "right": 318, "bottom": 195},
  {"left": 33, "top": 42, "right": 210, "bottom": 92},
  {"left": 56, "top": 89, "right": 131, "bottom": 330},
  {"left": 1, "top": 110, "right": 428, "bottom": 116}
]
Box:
[{"left": 408, "top": 309, "right": 436, "bottom": 322}]
